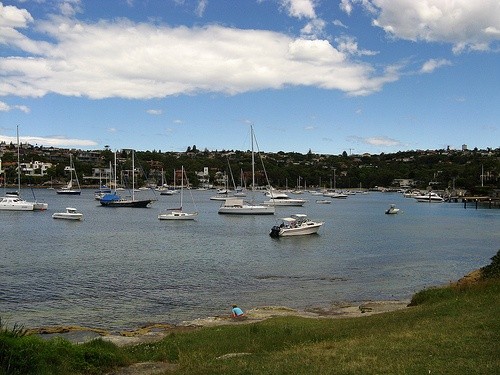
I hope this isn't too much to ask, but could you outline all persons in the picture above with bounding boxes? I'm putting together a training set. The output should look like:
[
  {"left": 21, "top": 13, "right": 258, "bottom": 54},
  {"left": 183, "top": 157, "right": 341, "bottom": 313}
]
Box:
[{"left": 232, "top": 304, "right": 244, "bottom": 317}]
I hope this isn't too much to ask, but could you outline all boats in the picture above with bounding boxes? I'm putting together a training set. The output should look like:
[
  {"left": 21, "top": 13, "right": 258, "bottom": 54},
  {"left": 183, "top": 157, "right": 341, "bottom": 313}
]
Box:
[
  {"left": 269, "top": 213, "right": 322, "bottom": 238},
  {"left": 51, "top": 207, "right": 83, "bottom": 220},
  {"left": 315, "top": 200, "right": 332, "bottom": 204},
  {"left": 99, "top": 150, "right": 421, "bottom": 208},
  {"left": 260, "top": 190, "right": 307, "bottom": 206},
  {"left": 413, "top": 192, "right": 444, "bottom": 203},
  {"left": 384, "top": 208, "right": 400, "bottom": 214}
]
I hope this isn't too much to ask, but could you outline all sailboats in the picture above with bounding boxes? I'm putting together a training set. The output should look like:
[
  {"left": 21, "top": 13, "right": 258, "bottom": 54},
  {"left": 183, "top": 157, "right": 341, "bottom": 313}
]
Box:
[
  {"left": 56, "top": 153, "right": 81, "bottom": 195},
  {"left": 217, "top": 125, "right": 275, "bottom": 215},
  {"left": 0, "top": 124, "right": 49, "bottom": 211},
  {"left": 157, "top": 165, "right": 199, "bottom": 220},
  {"left": 94, "top": 160, "right": 117, "bottom": 201}
]
